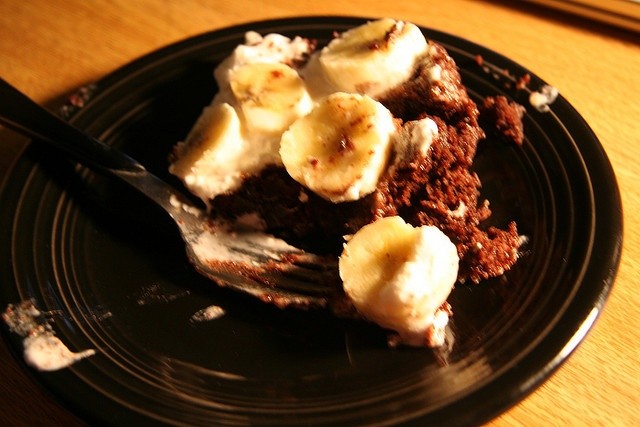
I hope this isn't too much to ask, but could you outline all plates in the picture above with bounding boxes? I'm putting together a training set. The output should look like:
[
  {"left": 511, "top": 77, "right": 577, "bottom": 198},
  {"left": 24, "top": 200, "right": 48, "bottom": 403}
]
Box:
[{"left": 1, "top": 12, "right": 626, "bottom": 426}]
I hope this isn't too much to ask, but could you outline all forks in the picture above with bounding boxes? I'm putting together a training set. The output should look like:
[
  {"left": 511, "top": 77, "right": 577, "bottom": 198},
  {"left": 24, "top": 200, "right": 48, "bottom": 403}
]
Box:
[{"left": 0, "top": 79, "right": 357, "bottom": 318}]
